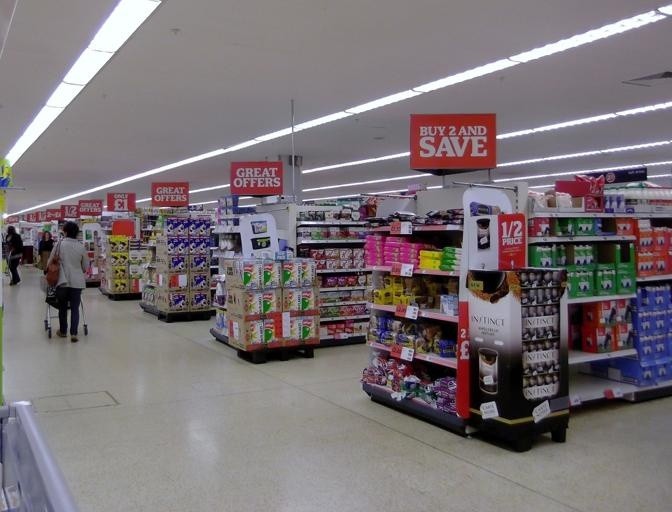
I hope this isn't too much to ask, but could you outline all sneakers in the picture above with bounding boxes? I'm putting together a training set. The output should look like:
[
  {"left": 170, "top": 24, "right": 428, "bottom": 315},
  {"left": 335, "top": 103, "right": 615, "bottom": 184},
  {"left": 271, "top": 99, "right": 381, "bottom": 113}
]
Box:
[
  {"left": 57, "top": 330, "right": 67, "bottom": 337},
  {"left": 71, "top": 335, "right": 79, "bottom": 342}
]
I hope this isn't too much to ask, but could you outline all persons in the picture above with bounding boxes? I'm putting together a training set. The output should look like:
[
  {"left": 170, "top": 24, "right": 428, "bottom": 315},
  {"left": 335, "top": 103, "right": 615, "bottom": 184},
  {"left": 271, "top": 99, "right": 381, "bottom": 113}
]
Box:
[
  {"left": 35, "top": 231, "right": 53, "bottom": 270},
  {"left": 44, "top": 221, "right": 89, "bottom": 342},
  {"left": 6, "top": 226, "right": 23, "bottom": 285}
]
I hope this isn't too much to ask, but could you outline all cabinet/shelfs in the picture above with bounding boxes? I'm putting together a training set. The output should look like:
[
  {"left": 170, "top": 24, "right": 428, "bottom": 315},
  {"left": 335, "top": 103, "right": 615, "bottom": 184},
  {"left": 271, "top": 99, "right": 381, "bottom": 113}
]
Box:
[
  {"left": 527, "top": 211, "right": 672, "bottom": 414},
  {"left": 361, "top": 181, "right": 528, "bottom": 452},
  {"left": 19, "top": 218, "right": 143, "bottom": 301},
  {"left": 139, "top": 207, "right": 215, "bottom": 321},
  {"left": 210, "top": 204, "right": 366, "bottom": 361}
]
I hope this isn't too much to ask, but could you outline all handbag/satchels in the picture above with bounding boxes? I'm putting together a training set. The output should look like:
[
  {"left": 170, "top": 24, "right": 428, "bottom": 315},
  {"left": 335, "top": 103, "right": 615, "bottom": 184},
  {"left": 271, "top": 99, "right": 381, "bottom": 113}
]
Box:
[
  {"left": 46, "top": 255, "right": 60, "bottom": 287},
  {"left": 37, "top": 260, "right": 44, "bottom": 270}
]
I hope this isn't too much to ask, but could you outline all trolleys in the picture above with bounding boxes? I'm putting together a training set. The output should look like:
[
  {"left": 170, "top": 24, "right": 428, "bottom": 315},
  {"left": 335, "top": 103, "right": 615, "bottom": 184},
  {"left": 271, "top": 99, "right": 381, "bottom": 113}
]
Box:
[
  {"left": 3, "top": 249, "right": 23, "bottom": 287},
  {"left": 39, "top": 275, "right": 88, "bottom": 340}
]
